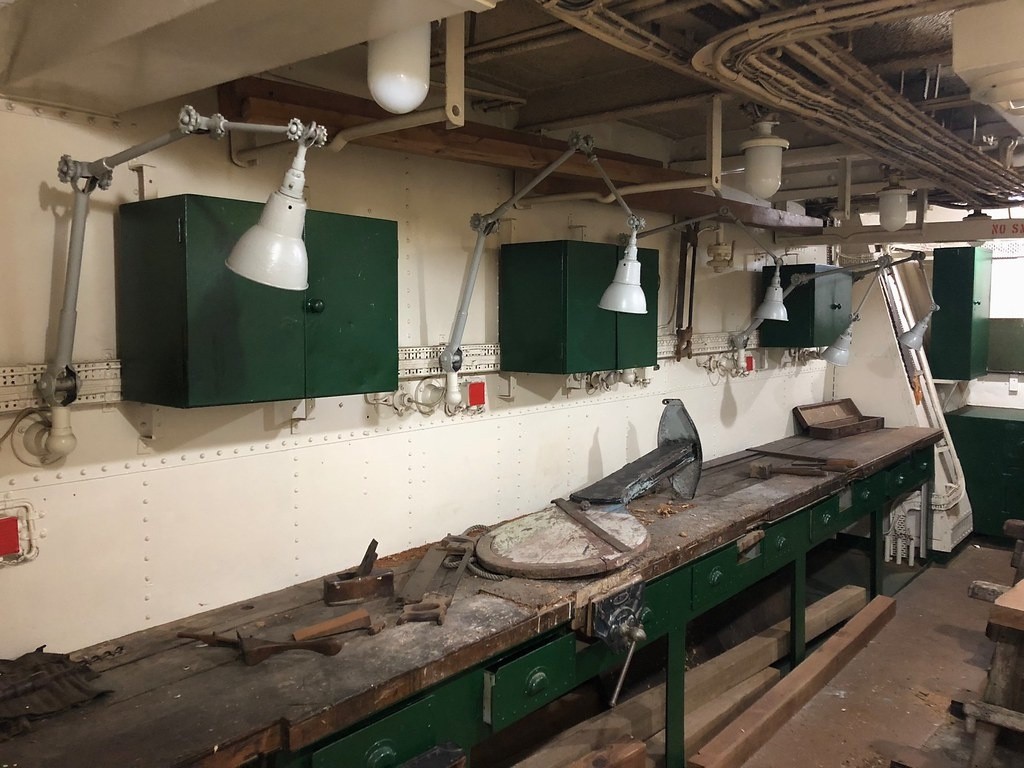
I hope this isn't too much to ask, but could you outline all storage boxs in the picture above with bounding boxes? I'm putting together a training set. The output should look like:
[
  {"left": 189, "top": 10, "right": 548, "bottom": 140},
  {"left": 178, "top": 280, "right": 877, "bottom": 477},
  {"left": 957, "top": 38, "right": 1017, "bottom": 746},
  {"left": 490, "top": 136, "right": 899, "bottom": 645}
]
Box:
[{"left": 792, "top": 398, "right": 884, "bottom": 439}]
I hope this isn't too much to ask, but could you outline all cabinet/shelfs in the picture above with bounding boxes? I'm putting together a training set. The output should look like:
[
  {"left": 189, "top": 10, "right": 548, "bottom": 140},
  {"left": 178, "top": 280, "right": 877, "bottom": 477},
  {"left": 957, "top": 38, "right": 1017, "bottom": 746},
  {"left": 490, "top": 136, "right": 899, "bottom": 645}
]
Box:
[
  {"left": 930, "top": 247, "right": 993, "bottom": 381},
  {"left": 118, "top": 193, "right": 399, "bottom": 408},
  {"left": 498, "top": 240, "right": 660, "bottom": 374},
  {"left": 759, "top": 264, "right": 853, "bottom": 347},
  {"left": 944, "top": 405, "right": 1024, "bottom": 540}
]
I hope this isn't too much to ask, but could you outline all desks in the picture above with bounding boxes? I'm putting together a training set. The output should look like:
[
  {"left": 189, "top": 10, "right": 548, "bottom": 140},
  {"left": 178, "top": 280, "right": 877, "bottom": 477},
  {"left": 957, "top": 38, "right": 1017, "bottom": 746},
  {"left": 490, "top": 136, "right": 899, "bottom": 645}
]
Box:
[{"left": 0, "top": 424, "right": 944, "bottom": 768}]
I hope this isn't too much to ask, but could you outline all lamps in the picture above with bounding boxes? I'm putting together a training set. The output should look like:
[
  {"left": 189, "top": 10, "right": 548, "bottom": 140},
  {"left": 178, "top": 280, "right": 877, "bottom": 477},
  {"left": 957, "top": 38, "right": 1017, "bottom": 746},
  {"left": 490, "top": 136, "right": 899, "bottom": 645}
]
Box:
[
  {"left": 616, "top": 206, "right": 789, "bottom": 323},
  {"left": 416, "top": 131, "right": 648, "bottom": 413},
  {"left": 963, "top": 209, "right": 992, "bottom": 247},
  {"left": 732, "top": 254, "right": 894, "bottom": 367},
  {"left": 366, "top": 18, "right": 442, "bottom": 114},
  {"left": 854, "top": 250, "right": 940, "bottom": 351},
  {"left": 875, "top": 167, "right": 914, "bottom": 231},
  {"left": 11, "top": 101, "right": 328, "bottom": 466},
  {"left": 737, "top": 102, "right": 790, "bottom": 201}
]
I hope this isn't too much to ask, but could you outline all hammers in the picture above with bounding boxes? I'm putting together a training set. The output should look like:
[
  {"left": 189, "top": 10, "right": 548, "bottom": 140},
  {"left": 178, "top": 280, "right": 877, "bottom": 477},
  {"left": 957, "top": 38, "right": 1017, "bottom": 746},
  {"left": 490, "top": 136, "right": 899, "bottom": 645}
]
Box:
[{"left": 748, "top": 461, "right": 829, "bottom": 480}]
